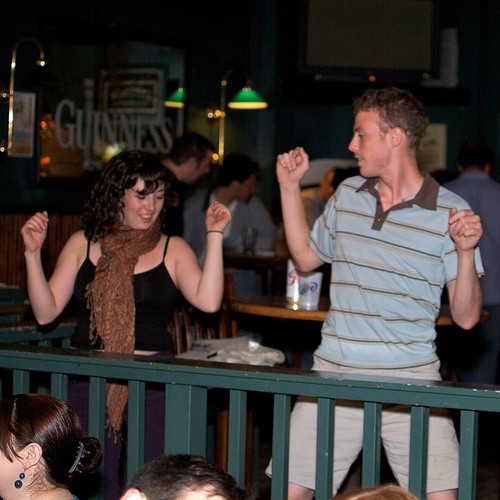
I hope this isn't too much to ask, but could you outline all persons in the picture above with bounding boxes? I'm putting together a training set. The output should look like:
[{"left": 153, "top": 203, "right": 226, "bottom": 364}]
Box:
[
  {"left": 298, "top": 167, "right": 351, "bottom": 235},
  {"left": 439, "top": 138, "right": 499, "bottom": 481},
  {"left": 275, "top": 85, "right": 487, "bottom": 499},
  {"left": 112, "top": 452, "right": 246, "bottom": 500},
  {"left": 17, "top": 148, "right": 232, "bottom": 488},
  {"left": 179, "top": 152, "right": 277, "bottom": 265},
  {"left": 153, "top": 130, "right": 220, "bottom": 241},
  {"left": 0, "top": 392, "right": 104, "bottom": 500}
]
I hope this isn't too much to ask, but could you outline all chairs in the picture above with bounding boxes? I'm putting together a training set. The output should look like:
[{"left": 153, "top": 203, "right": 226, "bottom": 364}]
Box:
[{"left": 167, "top": 271, "right": 250, "bottom": 475}]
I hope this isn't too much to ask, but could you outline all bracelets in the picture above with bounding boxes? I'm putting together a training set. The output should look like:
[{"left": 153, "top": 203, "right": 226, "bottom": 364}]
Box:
[{"left": 206, "top": 230, "right": 224, "bottom": 234}]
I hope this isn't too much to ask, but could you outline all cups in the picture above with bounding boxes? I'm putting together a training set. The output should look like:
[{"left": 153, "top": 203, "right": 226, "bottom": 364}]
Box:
[
  {"left": 297, "top": 271, "right": 324, "bottom": 312},
  {"left": 286, "top": 257, "right": 301, "bottom": 310}
]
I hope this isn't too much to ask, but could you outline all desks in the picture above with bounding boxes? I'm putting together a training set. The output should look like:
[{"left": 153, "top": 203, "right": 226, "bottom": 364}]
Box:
[{"left": 230, "top": 293, "right": 490, "bottom": 386}]
